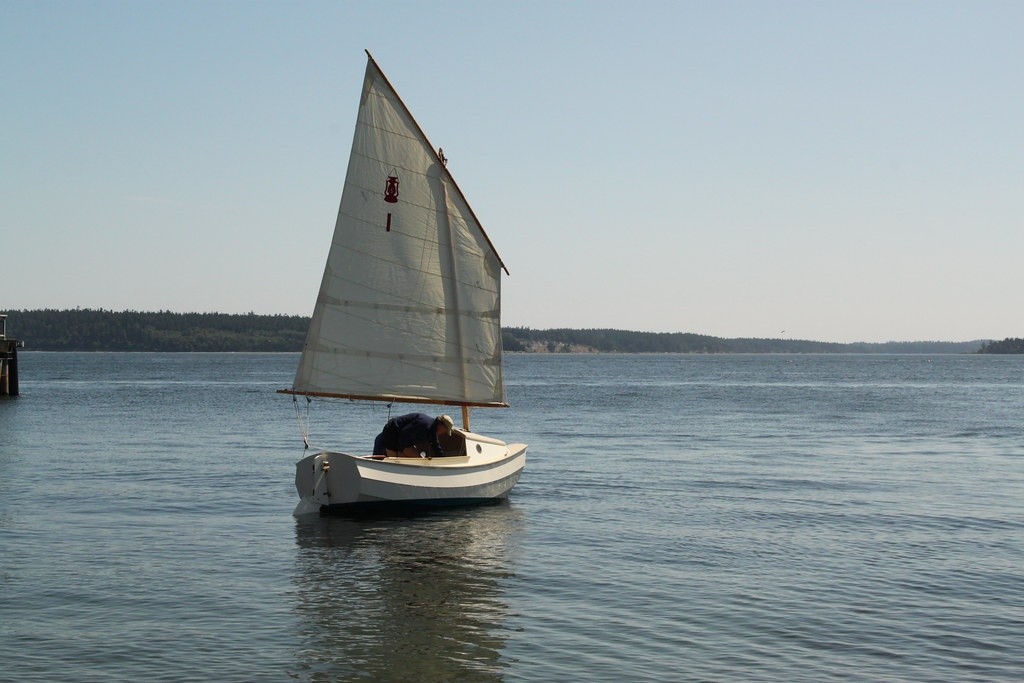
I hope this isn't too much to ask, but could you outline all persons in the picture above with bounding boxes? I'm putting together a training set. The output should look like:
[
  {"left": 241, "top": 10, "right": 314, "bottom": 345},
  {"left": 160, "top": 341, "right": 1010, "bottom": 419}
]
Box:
[{"left": 373, "top": 412, "right": 454, "bottom": 457}]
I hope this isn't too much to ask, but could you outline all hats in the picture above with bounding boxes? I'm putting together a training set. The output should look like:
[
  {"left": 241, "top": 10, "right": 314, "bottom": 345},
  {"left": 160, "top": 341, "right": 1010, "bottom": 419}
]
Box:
[{"left": 441, "top": 415, "right": 454, "bottom": 436}]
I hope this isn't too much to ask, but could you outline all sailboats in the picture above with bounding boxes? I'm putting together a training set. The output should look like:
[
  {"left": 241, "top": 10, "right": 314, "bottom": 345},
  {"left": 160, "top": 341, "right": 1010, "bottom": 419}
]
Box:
[{"left": 293, "top": 49, "right": 530, "bottom": 509}]
